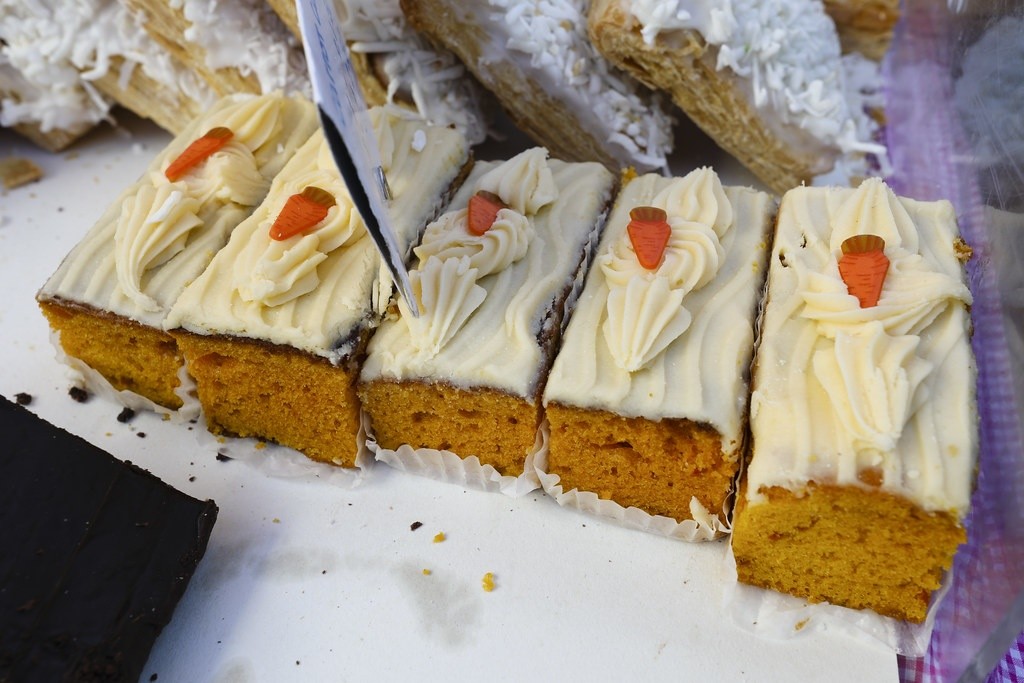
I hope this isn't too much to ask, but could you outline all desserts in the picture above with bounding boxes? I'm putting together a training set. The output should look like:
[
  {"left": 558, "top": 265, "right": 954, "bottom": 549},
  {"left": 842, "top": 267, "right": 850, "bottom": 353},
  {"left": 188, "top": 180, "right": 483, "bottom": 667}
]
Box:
[{"left": 39, "top": 97, "right": 977, "bottom": 632}]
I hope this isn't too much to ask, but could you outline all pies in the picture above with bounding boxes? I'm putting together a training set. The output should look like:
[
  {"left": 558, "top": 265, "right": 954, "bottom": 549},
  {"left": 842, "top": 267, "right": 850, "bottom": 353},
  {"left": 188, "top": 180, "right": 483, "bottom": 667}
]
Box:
[{"left": 1, "top": 0, "right": 895, "bottom": 204}]
